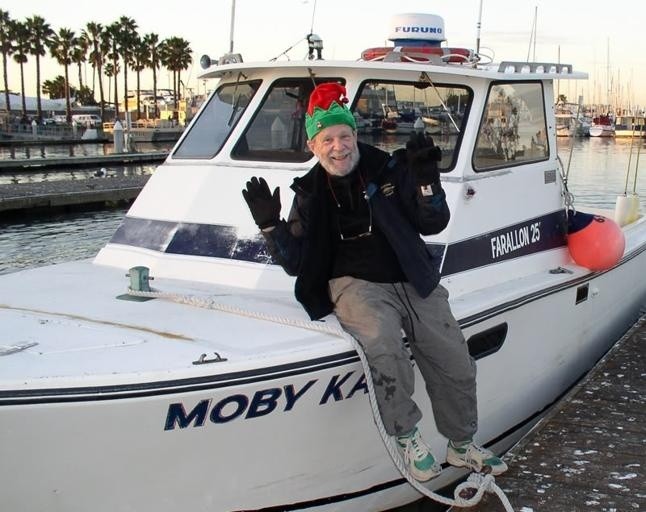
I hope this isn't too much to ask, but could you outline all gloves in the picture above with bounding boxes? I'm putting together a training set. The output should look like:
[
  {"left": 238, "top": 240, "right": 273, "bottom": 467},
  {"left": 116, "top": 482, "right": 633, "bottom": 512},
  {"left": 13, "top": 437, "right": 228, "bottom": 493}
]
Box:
[
  {"left": 243, "top": 177, "right": 282, "bottom": 230},
  {"left": 406, "top": 130, "right": 442, "bottom": 186}
]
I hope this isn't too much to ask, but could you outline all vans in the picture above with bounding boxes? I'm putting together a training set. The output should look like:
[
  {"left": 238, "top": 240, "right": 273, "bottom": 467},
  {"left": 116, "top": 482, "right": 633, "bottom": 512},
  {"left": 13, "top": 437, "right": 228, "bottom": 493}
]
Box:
[{"left": 70, "top": 114, "right": 103, "bottom": 127}]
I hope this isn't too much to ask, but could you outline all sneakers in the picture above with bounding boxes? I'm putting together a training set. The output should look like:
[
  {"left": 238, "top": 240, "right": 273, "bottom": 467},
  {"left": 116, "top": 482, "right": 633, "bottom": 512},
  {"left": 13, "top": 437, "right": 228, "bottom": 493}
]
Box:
[
  {"left": 396, "top": 426, "right": 442, "bottom": 481},
  {"left": 446, "top": 439, "right": 508, "bottom": 475}
]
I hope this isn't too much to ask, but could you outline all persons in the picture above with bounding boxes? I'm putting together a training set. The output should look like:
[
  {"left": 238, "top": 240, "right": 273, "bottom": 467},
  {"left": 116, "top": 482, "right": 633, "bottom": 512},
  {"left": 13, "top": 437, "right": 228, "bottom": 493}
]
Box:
[{"left": 241, "top": 81, "right": 512, "bottom": 482}]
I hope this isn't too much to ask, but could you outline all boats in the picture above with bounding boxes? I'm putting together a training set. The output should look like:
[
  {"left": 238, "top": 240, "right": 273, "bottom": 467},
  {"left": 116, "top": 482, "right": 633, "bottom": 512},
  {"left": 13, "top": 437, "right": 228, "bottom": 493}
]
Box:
[{"left": 0, "top": 1, "right": 646, "bottom": 511}]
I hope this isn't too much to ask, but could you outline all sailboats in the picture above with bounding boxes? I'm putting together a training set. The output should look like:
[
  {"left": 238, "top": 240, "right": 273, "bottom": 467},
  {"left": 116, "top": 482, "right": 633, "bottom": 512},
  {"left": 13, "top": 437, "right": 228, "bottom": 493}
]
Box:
[
  {"left": 350, "top": 81, "right": 464, "bottom": 137},
  {"left": 547, "top": 37, "right": 646, "bottom": 138}
]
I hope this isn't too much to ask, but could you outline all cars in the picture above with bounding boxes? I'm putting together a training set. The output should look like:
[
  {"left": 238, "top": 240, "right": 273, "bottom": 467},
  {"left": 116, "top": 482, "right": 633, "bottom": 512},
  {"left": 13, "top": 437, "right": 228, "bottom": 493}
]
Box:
[{"left": 13, "top": 114, "right": 76, "bottom": 126}]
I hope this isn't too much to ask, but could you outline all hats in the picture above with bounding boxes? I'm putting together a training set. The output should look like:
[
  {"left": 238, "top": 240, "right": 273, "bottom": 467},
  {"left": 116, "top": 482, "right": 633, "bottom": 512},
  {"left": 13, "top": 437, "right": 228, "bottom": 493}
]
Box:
[{"left": 305, "top": 82, "right": 356, "bottom": 141}]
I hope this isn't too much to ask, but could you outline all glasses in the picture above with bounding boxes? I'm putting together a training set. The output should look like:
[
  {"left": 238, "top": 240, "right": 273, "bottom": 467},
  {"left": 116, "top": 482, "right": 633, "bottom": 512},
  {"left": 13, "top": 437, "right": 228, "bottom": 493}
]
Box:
[{"left": 337, "top": 195, "right": 375, "bottom": 244}]
